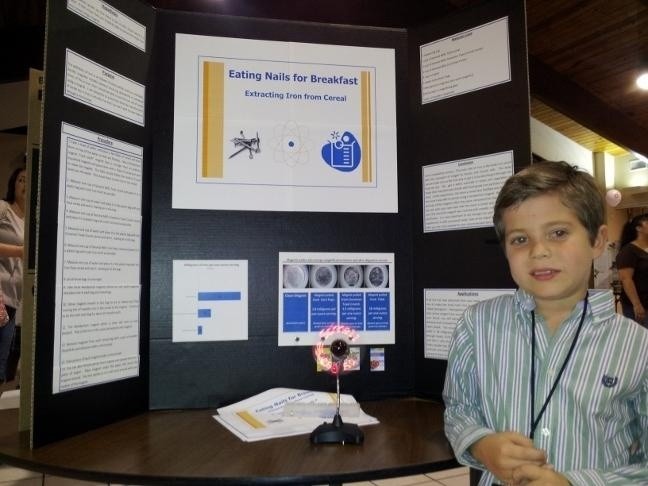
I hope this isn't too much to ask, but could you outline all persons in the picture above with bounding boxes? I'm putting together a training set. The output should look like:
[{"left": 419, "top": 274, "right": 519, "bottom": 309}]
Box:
[
  {"left": 0, "top": 166, "right": 27, "bottom": 385},
  {"left": 441, "top": 160, "right": 647, "bottom": 485},
  {"left": 616, "top": 213, "right": 647, "bottom": 326}
]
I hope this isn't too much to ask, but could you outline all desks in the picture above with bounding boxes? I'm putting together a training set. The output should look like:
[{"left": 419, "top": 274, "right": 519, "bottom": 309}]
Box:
[{"left": 0, "top": 398, "right": 461, "bottom": 486}]
